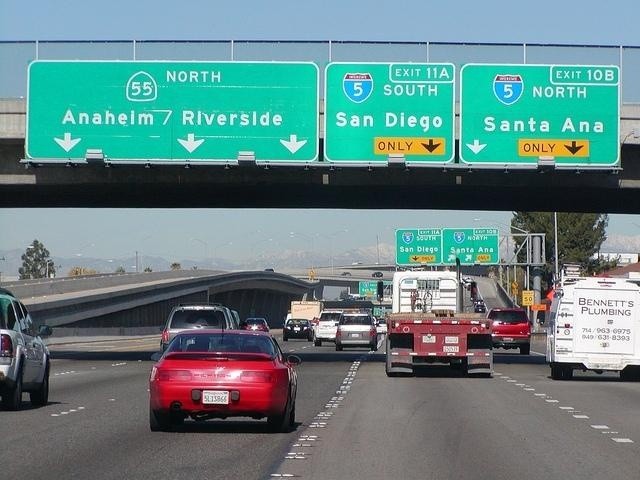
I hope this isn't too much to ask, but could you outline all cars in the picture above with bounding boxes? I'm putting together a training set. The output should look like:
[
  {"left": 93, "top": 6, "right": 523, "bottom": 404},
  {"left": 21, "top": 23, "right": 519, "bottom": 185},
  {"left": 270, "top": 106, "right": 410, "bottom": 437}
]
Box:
[
  {"left": 150, "top": 301, "right": 387, "bottom": 433},
  {"left": 487, "top": 307, "right": 531, "bottom": 353},
  {"left": 475, "top": 299, "right": 485, "bottom": 313},
  {"left": 372, "top": 272, "right": 383, "bottom": 277},
  {"left": 0, "top": 287, "right": 51, "bottom": 411}
]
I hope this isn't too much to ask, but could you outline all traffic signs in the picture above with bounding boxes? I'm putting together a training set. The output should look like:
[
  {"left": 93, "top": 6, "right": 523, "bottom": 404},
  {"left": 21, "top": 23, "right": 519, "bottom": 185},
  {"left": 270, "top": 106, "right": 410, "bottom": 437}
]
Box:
[
  {"left": 521, "top": 291, "right": 534, "bottom": 307},
  {"left": 396, "top": 228, "right": 498, "bottom": 266}
]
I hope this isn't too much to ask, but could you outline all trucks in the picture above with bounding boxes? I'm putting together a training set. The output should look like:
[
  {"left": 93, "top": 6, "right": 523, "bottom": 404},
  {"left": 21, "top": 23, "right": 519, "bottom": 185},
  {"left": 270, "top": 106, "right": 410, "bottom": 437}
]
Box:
[
  {"left": 546, "top": 276, "right": 639, "bottom": 380},
  {"left": 387, "top": 272, "right": 492, "bottom": 377}
]
